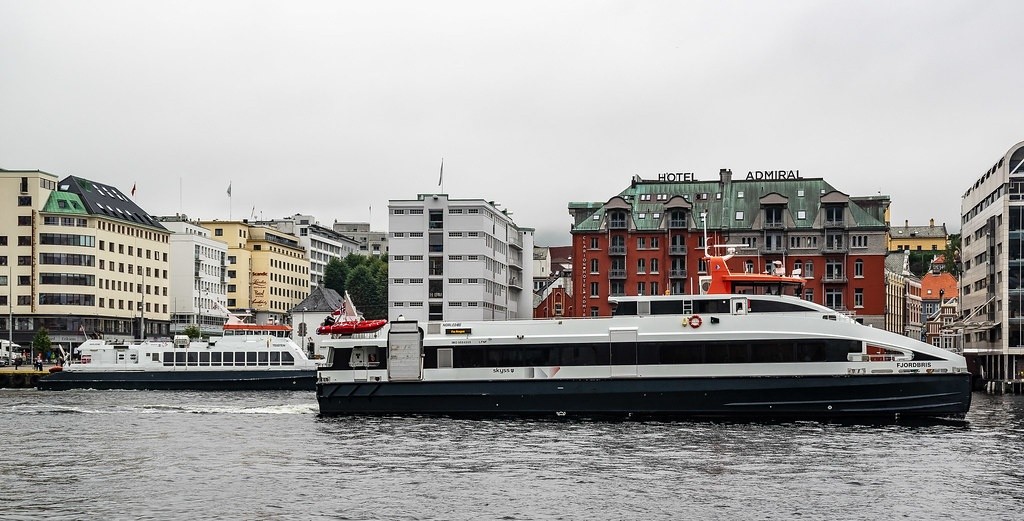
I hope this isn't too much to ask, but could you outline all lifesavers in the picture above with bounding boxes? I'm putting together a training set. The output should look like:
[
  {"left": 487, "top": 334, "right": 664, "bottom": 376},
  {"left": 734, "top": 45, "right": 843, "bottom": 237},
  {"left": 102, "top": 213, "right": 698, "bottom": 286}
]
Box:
[{"left": 688, "top": 314, "right": 703, "bottom": 329}]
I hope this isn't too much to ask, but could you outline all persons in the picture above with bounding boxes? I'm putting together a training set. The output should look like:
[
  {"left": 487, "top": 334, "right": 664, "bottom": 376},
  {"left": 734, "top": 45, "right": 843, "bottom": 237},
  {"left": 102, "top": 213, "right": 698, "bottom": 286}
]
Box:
[
  {"left": 15, "top": 355, "right": 19, "bottom": 370},
  {"left": 38, "top": 353, "right": 43, "bottom": 371}
]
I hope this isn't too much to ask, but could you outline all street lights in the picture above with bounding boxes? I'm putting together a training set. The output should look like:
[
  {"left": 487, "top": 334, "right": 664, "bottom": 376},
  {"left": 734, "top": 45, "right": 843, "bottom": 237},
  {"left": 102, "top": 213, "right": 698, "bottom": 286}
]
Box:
[
  {"left": 139, "top": 305, "right": 145, "bottom": 344},
  {"left": 195, "top": 276, "right": 203, "bottom": 341},
  {"left": 302, "top": 306, "right": 308, "bottom": 351}
]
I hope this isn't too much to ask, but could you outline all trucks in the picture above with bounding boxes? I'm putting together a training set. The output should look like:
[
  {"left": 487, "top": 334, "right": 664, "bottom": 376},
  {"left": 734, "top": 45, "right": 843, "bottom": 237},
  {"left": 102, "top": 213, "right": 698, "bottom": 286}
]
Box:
[{"left": 0, "top": 339, "right": 26, "bottom": 364}]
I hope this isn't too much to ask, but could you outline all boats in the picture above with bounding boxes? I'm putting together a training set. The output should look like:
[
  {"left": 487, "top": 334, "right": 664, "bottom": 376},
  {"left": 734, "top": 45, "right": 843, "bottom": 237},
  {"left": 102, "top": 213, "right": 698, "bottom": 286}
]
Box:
[
  {"left": 316, "top": 291, "right": 387, "bottom": 335},
  {"left": 315, "top": 208, "right": 973, "bottom": 422},
  {"left": 38, "top": 295, "right": 317, "bottom": 392}
]
